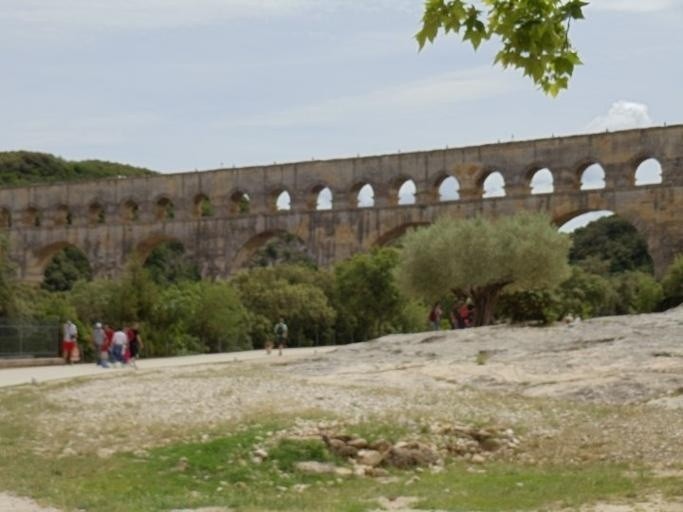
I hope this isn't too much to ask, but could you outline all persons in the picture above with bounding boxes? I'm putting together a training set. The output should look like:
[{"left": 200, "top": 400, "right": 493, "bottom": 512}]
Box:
[
  {"left": 61, "top": 321, "right": 77, "bottom": 364},
  {"left": 92, "top": 321, "right": 145, "bottom": 367},
  {"left": 274, "top": 316, "right": 288, "bottom": 354},
  {"left": 430, "top": 302, "right": 444, "bottom": 329},
  {"left": 260, "top": 325, "right": 277, "bottom": 355},
  {"left": 447, "top": 297, "right": 478, "bottom": 331}
]
